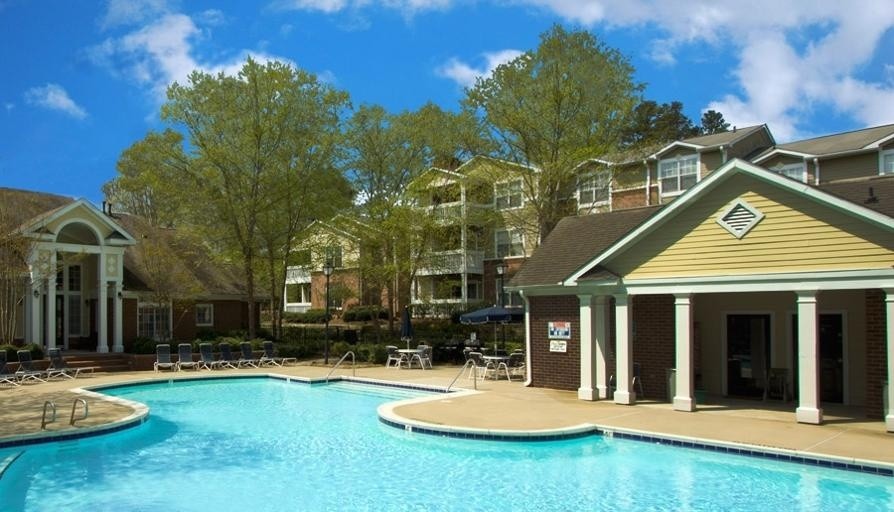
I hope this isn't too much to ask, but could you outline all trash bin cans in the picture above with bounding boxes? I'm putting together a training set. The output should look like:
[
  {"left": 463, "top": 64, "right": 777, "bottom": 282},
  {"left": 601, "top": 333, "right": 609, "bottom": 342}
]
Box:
[{"left": 665, "top": 368, "right": 676, "bottom": 403}]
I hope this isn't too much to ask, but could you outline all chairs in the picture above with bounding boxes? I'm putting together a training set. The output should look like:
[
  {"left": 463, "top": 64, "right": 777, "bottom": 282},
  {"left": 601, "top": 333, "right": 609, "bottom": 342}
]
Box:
[
  {"left": 608, "top": 361, "right": 644, "bottom": 398},
  {"left": 385, "top": 343, "right": 432, "bottom": 370},
  {"left": 0, "top": 348, "right": 94, "bottom": 388},
  {"left": 152, "top": 341, "right": 297, "bottom": 373},
  {"left": 463, "top": 346, "right": 525, "bottom": 383}
]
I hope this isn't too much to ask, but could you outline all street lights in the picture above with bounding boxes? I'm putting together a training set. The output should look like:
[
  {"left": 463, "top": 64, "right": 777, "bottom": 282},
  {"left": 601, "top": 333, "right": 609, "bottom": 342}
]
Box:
[
  {"left": 495, "top": 263, "right": 510, "bottom": 364},
  {"left": 322, "top": 262, "right": 334, "bottom": 364}
]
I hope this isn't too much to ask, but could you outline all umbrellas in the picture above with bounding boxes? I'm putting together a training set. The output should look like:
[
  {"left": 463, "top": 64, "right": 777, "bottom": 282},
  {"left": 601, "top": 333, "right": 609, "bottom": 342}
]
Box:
[
  {"left": 400, "top": 304, "right": 416, "bottom": 349},
  {"left": 459, "top": 304, "right": 525, "bottom": 355}
]
